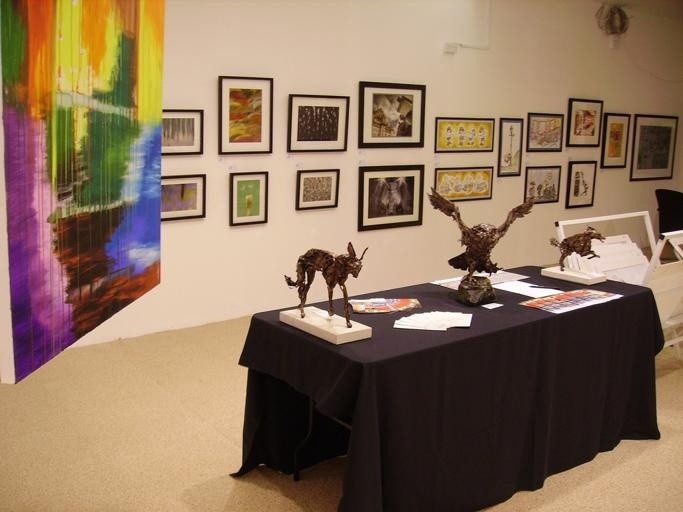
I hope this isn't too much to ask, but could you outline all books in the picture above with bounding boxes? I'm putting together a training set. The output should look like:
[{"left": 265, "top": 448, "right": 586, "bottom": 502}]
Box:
[
  {"left": 393, "top": 310, "right": 473, "bottom": 331},
  {"left": 347, "top": 296, "right": 421, "bottom": 314}
]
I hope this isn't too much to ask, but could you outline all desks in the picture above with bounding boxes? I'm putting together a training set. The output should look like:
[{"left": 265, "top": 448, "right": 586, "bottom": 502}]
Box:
[{"left": 250, "top": 265, "right": 652, "bottom": 512}]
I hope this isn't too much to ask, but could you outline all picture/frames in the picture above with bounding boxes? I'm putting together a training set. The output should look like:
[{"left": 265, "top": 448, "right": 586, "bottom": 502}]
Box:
[
  {"left": 629, "top": 114, "right": 679, "bottom": 182},
  {"left": 526, "top": 110, "right": 565, "bottom": 153},
  {"left": 565, "top": 159, "right": 598, "bottom": 209},
  {"left": 160, "top": 173, "right": 208, "bottom": 222},
  {"left": 599, "top": 111, "right": 631, "bottom": 169},
  {"left": 497, "top": 117, "right": 524, "bottom": 177},
  {"left": 229, "top": 171, "right": 269, "bottom": 226},
  {"left": 566, "top": 97, "right": 604, "bottom": 148},
  {"left": 358, "top": 82, "right": 427, "bottom": 148},
  {"left": 218, "top": 75, "right": 274, "bottom": 155},
  {"left": 161, "top": 109, "right": 205, "bottom": 156},
  {"left": 295, "top": 168, "right": 341, "bottom": 210},
  {"left": 434, "top": 165, "right": 495, "bottom": 203},
  {"left": 358, "top": 164, "right": 427, "bottom": 231},
  {"left": 524, "top": 165, "right": 562, "bottom": 205},
  {"left": 286, "top": 93, "right": 350, "bottom": 153},
  {"left": 433, "top": 116, "right": 496, "bottom": 153}
]
